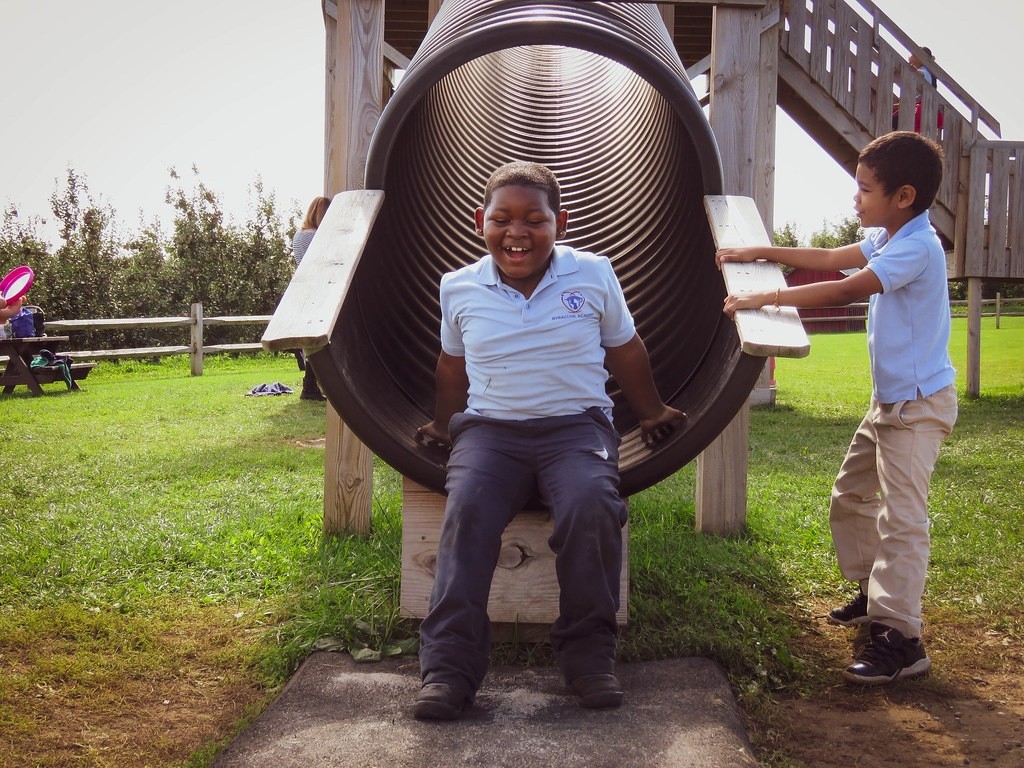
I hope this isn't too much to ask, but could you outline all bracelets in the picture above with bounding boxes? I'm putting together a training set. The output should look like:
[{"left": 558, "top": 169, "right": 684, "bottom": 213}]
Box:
[{"left": 775, "top": 287, "right": 780, "bottom": 308}]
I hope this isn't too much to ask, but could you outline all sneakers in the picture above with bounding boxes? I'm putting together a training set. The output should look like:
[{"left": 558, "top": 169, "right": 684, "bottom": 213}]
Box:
[
  {"left": 828, "top": 592, "right": 869, "bottom": 624},
  {"left": 844, "top": 624, "right": 930, "bottom": 683}
]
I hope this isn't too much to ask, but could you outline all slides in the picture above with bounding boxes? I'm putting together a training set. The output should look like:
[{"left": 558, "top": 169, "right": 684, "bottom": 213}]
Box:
[{"left": 260, "top": 0, "right": 811, "bottom": 499}]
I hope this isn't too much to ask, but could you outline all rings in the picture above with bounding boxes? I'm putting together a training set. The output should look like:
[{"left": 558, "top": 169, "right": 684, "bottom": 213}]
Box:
[{"left": 418, "top": 427, "right": 423, "bottom": 431}]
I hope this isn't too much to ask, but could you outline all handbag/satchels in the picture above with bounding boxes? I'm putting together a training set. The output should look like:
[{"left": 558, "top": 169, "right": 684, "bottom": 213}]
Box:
[{"left": 9, "top": 306, "right": 46, "bottom": 337}]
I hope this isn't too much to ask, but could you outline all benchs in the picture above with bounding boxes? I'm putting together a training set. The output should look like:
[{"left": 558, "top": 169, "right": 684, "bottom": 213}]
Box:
[{"left": 28, "top": 362, "right": 98, "bottom": 380}]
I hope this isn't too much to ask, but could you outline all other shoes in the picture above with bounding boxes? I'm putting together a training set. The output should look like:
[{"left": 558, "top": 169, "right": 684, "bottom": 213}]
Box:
[
  {"left": 413, "top": 682, "right": 475, "bottom": 718},
  {"left": 300, "top": 392, "right": 327, "bottom": 401},
  {"left": 572, "top": 672, "right": 623, "bottom": 709}
]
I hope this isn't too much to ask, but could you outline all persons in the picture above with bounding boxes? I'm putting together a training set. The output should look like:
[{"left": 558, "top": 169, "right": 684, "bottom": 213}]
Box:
[
  {"left": 0, "top": 293, "right": 26, "bottom": 325},
  {"left": 714, "top": 130, "right": 958, "bottom": 686},
  {"left": 891, "top": 46, "right": 945, "bottom": 134},
  {"left": 291, "top": 197, "right": 330, "bottom": 402},
  {"left": 409, "top": 162, "right": 689, "bottom": 721}
]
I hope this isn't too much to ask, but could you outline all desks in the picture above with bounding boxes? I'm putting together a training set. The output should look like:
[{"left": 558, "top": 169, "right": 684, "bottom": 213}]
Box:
[{"left": 0, "top": 336, "right": 83, "bottom": 396}]
